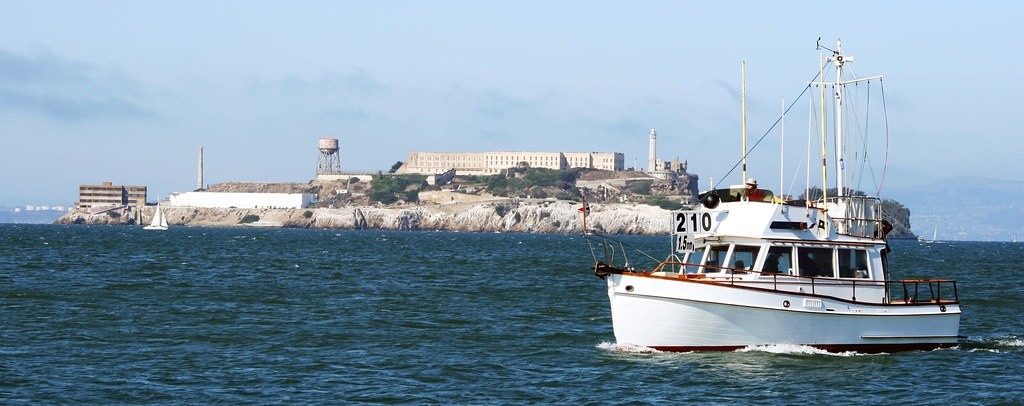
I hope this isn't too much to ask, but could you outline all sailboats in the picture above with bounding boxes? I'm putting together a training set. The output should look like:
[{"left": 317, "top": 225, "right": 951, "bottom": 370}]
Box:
[{"left": 141, "top": 195, "right": 169, "bottom": 230}]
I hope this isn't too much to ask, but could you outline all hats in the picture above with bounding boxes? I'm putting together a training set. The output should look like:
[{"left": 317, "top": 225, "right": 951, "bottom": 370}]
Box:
[{"left": 746, "top": 178, "right": 758, "bottom": 186}]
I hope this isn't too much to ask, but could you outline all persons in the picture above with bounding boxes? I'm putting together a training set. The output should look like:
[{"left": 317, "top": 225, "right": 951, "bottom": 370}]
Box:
[
  {"left": 761, "top": 250, "right": 783, "bottom": 275},
  {"left": 734, "top": 260, "right": 744, "bottom": 268},
  {"left": 745, "top": 178, "right": 758, "bottom": 189}
]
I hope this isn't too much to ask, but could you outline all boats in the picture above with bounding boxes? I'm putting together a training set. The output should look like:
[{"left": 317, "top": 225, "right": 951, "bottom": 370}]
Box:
[{"left": 579, "top": 34, "right": 964, "bottom": 354}]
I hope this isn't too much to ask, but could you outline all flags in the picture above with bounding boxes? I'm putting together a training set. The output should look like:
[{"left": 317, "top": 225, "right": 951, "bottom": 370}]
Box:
[{"left": 578, "top": 200, "right": 590, "bottom": 217}]
